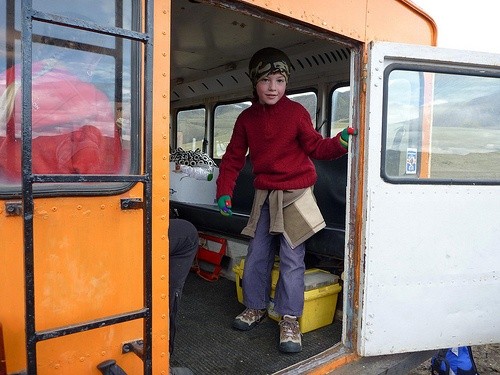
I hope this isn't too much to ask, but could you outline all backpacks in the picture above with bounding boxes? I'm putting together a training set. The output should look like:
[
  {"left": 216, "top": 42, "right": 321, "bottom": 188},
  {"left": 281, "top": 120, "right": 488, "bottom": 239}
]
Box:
[{"left": 432, "top": 346, "right": 477, "bottom": 375}]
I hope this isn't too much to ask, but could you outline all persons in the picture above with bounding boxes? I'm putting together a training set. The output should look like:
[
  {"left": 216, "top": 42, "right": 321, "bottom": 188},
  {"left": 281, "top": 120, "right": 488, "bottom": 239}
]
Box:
[
  {"left": 0, "top": 8, "right": 201, "bottom": 363},
  {"left": 212, "top": 45, "right": 359, "bottom": 355}
]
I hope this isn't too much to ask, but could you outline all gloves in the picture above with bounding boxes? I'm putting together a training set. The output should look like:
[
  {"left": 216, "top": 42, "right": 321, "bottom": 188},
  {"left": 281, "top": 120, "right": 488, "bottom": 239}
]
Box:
[
  {"left": 340, "top": 127, "right": 358, "bottom": 148},
  {"left": 218, "top": 195, "right": 232, "bottom": 216}
]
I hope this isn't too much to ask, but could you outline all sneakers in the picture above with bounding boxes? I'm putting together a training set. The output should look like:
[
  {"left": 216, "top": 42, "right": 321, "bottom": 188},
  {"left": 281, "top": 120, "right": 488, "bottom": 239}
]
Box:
[
  {"left": 279, "top": 315, "right": 302, "bottom": 352},
  {"left": 232, "top": 307, "right": 266, "bottom": 329}
]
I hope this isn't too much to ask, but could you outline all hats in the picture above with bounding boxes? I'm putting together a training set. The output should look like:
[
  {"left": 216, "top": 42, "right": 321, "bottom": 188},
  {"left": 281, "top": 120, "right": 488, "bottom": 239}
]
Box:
[{"left": 248, "top": 47, "right": 294, "bottom": 83}]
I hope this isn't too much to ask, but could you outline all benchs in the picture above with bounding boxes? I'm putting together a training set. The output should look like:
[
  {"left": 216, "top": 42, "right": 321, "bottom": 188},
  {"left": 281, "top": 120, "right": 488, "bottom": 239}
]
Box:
[{"left": 169, "top": 147, "right": 402, "bottom": 263}]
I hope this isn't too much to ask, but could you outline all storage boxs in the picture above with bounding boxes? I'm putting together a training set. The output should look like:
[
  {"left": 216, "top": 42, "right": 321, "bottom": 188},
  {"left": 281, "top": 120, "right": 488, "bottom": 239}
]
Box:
[{"left": 231, "top": 253, "right": 343, "bottom": 333}]
